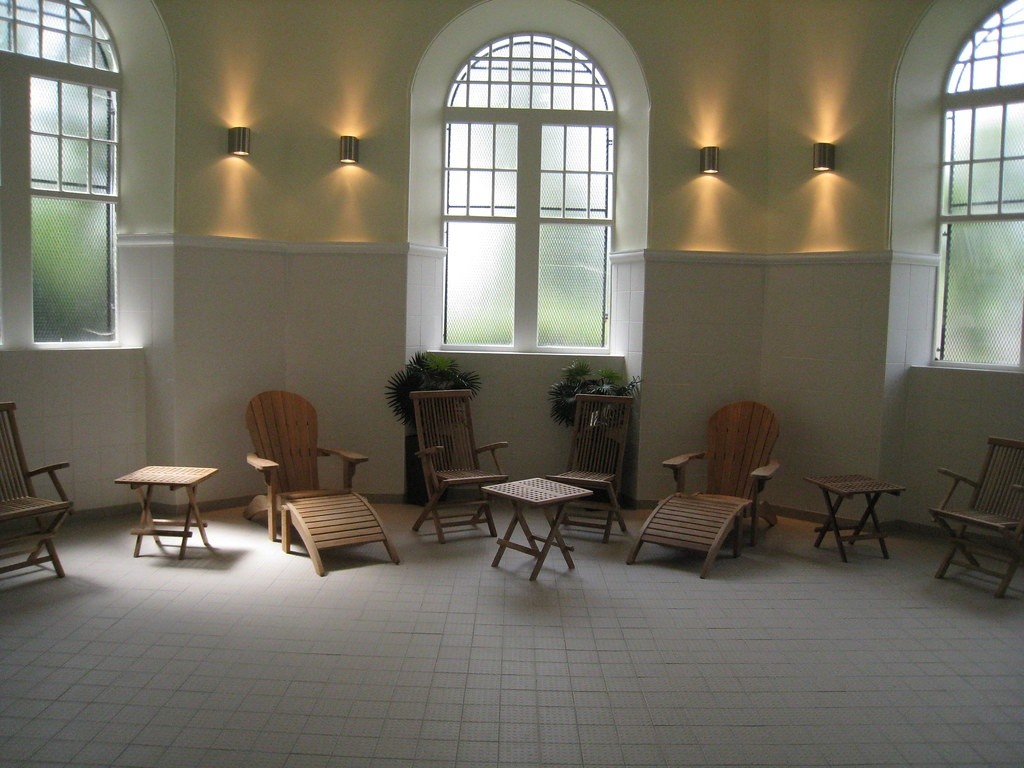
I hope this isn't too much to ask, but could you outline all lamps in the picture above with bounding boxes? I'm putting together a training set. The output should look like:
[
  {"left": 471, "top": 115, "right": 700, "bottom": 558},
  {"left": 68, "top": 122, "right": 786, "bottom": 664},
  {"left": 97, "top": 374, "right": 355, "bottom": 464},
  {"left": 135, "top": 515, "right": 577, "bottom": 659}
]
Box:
[
  {"left": 339, "top": 136, "right": 359, "bottom": 164},
  {"left": 227, "top": 127, "right": 250, "bottom": 156},
  {"left": 813, "top": 143, "right": 835, "bottom": 172},
  {"left": 700, "top": 147, "right": 719, "bottom": 174}
]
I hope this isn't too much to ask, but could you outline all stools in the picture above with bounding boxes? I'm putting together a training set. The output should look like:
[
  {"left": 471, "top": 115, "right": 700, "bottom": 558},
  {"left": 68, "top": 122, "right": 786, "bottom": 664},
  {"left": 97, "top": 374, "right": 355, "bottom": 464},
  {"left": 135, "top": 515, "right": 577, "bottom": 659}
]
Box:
[
  {"left": 115, "top": 465, "right": 219, "bottom": 560},
  {"left": 802, "top": 474, "right": 906, "bottom": 563},
  {"left": 481, "top": 478, "right": 593, "bottom": 582}
]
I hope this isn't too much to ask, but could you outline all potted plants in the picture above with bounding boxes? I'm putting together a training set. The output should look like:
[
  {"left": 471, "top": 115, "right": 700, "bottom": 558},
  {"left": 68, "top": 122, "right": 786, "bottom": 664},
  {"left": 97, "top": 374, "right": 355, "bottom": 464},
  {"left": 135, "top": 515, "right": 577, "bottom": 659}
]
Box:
[
  {"left": 385, "top": 350, "right": 482, "bottom": 506},
  {"left": 546, "top": 357, "right": 647, "bottom": 512}
]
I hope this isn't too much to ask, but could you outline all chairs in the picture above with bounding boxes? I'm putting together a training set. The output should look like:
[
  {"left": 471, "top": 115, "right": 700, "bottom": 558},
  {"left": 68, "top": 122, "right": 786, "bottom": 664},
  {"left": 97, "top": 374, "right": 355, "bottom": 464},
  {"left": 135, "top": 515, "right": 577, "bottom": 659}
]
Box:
[
  {"left": 409, "top": 389, "right": 510, "bottom": 543},
  {"left": 546, "top": 394, "right": 636, "bottom": 543},
  {"left": 245, "top": 390, "right": 400, "bottom": 577},
  {"left": 0, "top": 402, "right": 74, "bottom": 578},
  {"left": 928, "top": 436, "right": 1024, "bottom": 598},
  {"left": 625, "top": 400, "right": 780, "bottom": 579}
]
